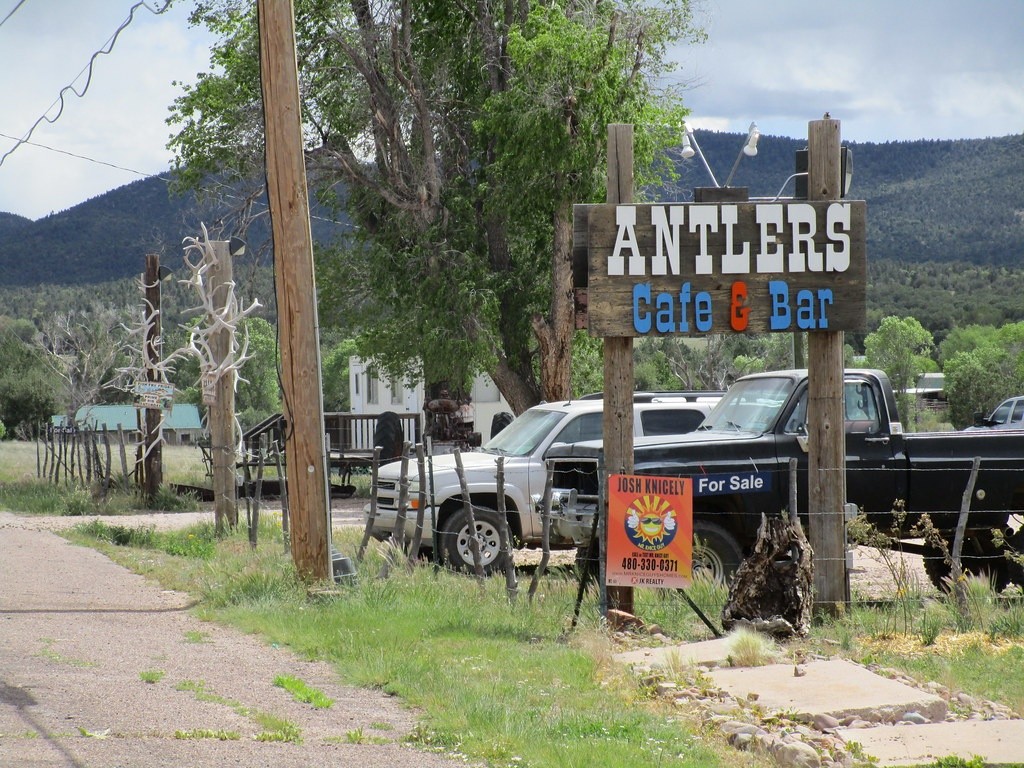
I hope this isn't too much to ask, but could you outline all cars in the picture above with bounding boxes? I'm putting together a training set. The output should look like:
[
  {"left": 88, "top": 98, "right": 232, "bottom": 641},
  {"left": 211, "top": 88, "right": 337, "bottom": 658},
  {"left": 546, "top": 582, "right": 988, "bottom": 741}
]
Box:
[{"left": 963, "top": 394, "right": 1024, "bottom": 433}]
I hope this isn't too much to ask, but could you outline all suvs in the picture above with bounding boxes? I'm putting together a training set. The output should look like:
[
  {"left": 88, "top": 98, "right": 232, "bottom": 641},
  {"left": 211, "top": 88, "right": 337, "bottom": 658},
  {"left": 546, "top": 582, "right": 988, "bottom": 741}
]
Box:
[{"left": 362, "top": 389, "right": 765, "bottom": 576}]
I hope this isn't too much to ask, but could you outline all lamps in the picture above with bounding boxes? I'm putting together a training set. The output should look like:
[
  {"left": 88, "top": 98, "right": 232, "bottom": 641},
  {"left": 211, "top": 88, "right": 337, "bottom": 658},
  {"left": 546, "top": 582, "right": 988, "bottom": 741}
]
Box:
[
  {"left": 679, "top": 119, "right": 719, "bottom": 187},
  {"left": 725, "top": 119, "right": 759, "bottom": 185}
]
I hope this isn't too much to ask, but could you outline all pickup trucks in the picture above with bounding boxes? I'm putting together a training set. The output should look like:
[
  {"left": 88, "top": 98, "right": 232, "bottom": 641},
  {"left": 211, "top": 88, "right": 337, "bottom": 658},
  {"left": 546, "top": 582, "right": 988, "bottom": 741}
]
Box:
[{"left": 545, "top": 366, "right": 1024, "bottom": 617}]
